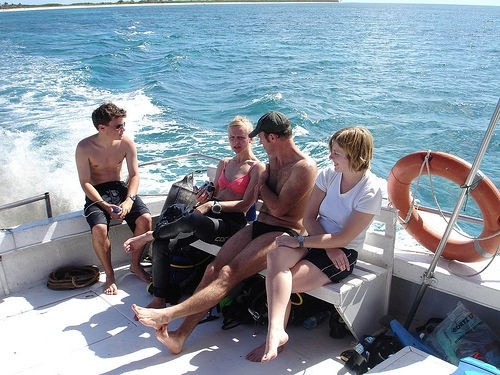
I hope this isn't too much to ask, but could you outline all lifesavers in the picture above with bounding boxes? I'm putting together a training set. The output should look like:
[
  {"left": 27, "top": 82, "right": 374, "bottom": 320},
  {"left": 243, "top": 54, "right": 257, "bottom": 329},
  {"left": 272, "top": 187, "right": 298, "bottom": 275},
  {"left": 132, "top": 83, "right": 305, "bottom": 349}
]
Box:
[{"left": 387, "top": 150, "right": 500, "bottom": 262}]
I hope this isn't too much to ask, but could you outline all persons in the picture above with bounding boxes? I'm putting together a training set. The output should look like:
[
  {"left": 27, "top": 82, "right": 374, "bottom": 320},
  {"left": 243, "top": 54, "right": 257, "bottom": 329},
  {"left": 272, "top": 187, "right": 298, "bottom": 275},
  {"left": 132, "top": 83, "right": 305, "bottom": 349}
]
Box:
[
  {"left": 75, "top": 102, "right": 152, "bottom": 295},
  {"left": 246, "top": 126, "right": 381, "bottom": 363},
  {"left": 131, "top": 111, "right": 318, "bottom": 355},
  {"left": 122, "top": 116, "right": 266, "bottom": 321}
]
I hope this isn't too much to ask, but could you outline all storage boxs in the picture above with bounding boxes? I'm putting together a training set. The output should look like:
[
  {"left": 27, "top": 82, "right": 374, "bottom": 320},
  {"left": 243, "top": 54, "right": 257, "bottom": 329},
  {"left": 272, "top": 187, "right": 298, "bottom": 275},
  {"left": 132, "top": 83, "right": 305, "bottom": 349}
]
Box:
[{"left": 362, "top": 346, "right": 458, "bottom": 375}]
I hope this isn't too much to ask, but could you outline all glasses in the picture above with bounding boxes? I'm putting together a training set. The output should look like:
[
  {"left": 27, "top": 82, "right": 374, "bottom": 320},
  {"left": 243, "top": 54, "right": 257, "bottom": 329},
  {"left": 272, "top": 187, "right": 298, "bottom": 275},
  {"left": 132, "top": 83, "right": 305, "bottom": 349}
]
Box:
[{"left": 107, "top": 122, "right": 125, "bottom": 131}]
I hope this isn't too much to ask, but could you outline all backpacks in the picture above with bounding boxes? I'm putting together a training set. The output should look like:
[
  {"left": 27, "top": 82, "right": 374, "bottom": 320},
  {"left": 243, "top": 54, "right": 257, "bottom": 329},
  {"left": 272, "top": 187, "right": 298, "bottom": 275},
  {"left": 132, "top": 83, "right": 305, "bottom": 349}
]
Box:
[{"left": 215, "top": 278, "right": 321, "bottom": 330}]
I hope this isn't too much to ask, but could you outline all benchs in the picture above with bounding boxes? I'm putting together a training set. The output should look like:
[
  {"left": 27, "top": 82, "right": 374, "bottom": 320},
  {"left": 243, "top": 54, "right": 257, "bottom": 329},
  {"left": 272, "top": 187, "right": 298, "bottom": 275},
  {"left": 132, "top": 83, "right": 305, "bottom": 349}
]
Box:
[{"left": 188, "top": 164, "right": 398, "bottom": 343}]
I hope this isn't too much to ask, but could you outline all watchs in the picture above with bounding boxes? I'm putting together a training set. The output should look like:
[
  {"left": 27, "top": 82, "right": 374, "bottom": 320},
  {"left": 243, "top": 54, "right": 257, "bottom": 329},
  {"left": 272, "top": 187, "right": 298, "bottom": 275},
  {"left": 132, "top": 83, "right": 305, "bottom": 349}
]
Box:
[
  {"left": 211, "top": 200, "right": 222, "bottom": 214},
  {"left": 127, "top": 193, "right": 136, "bottom": 201},
  {"left": 296, "top": 235, "right": 305, "bottom": 248}
]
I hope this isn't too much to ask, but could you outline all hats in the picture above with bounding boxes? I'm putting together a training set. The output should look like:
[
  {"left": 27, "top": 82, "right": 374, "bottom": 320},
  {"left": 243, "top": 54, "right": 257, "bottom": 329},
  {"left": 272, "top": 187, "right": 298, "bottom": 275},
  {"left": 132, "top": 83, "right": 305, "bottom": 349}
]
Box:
[{"left": 247, "top": 111, "right": 292, "bottom": 138}]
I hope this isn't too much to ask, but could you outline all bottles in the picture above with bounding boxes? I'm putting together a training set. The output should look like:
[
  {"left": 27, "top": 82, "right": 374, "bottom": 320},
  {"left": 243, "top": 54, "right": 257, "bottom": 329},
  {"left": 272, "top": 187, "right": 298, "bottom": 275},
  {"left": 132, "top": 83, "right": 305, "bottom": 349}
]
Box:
[{"left": 305, "top": 310, "right": 328, "bottom": 328}]
